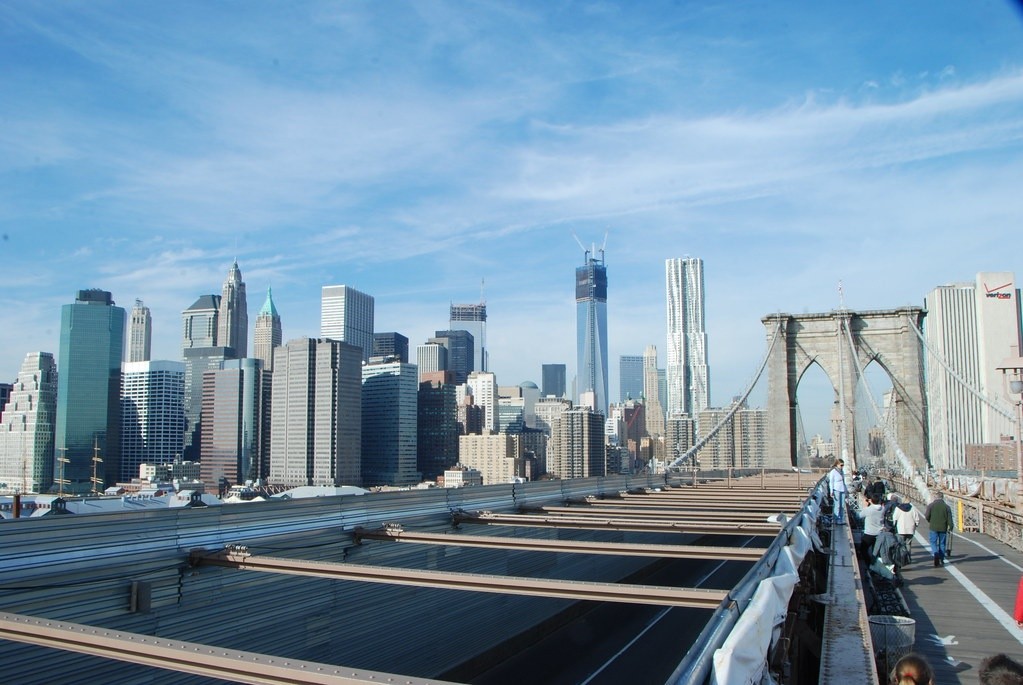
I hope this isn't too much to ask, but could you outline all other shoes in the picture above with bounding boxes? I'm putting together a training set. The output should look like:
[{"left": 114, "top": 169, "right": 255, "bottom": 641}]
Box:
[
  {"left": 835, "top": 518, "right": 847, "bottom": 525},
  {"left": 934, "top": 552, "right": 940, "bottom": 566}
]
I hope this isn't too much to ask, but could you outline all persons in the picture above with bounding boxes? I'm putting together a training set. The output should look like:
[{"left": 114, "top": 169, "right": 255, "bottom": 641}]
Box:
[
  {"left": 864, "top": 476, "right": 885, "bottom": 507},
  {"left": 850, "top": 467, "right": 867, "bottom": 492},
  {"left": 829, "top": 459, "right": 849, "bottom": 525},
  {"left": 889, "top": 654, "right": 934, "bottom": 685},
  {"left": 924, "top": 492, "right": 954, "bottom": 567},
  {"left": 979, "top": 654, "right": 1023, "bottom": 685},
  {"left": 893, "top": 495, "right": 920, "bottom": 564},
  {"left": 859, "top": 494, "right": 886, "bottom": 557}
]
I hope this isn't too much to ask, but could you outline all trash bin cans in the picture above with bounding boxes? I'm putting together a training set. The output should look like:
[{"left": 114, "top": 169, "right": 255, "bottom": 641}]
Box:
[{"left": 868, "top": 614, "right": 916, "bottom": 685}]
